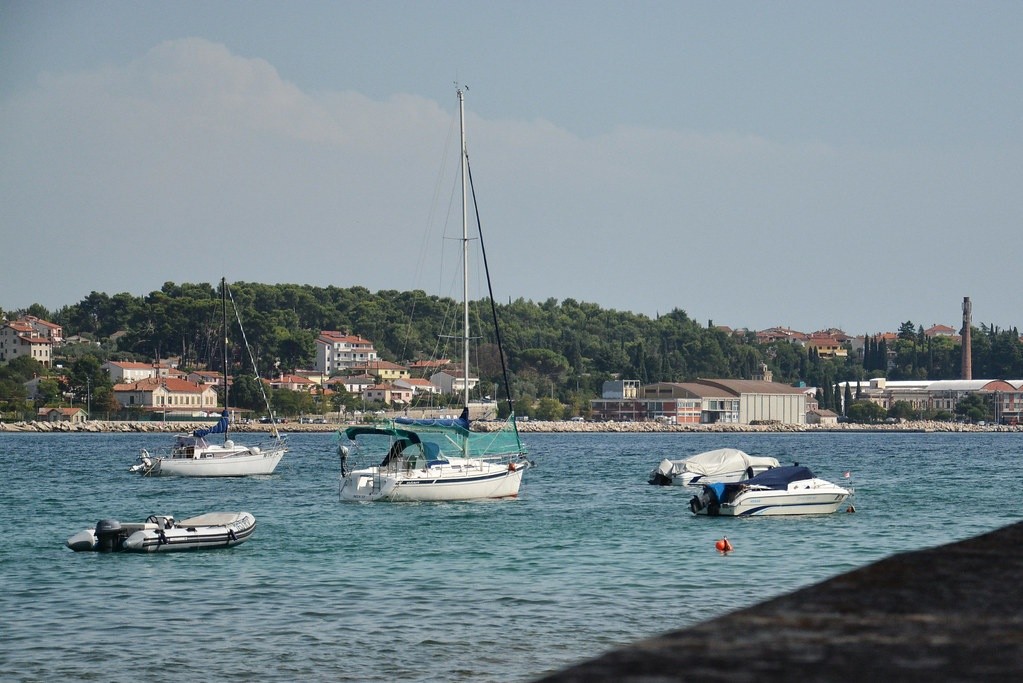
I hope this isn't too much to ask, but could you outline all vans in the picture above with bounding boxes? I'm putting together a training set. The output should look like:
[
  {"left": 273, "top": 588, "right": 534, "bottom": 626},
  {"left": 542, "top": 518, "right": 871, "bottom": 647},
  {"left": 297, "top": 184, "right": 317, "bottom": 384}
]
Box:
[{"left": 570, "top": 417, "right": 584, "bottom": 422}]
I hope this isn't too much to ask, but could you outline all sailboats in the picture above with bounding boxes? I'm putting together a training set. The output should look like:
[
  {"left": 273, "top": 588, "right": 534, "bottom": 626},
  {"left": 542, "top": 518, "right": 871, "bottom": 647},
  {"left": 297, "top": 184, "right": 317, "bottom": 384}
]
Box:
[
  {"left": 337, "top": 80, "right": 536, "bottom": 503},
  {"left": 129, "top": 274, "right": 288, "bottom": 477}
]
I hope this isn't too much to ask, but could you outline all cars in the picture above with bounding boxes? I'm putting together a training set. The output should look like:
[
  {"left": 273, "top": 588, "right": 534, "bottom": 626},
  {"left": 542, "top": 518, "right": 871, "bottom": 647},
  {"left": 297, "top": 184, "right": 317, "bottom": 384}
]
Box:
[
  {"left": 298, "top": 417, "right": 327, "bottom": 424},
  {"left": 586, "top": 419, "right": 605, "bottom": 424},
  {"left": 352, "top": 409, "right": 385, "bottom": 415},
  {"left": 259, "top": 416, "right": 288, "bottom": 423}
]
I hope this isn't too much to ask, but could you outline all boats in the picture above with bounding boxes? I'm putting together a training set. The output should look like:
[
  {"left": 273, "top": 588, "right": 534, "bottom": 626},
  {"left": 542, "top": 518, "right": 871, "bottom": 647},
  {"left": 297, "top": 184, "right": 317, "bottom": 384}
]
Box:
[
  {"left": 648, "top": 449, "right": 782, "bottom": 486},
  {"left": 66, "top": 512, "right": 257, "bottom": 554},
  {"left": 688, "top": 461, "right": 855, "bottom": 516}
]
